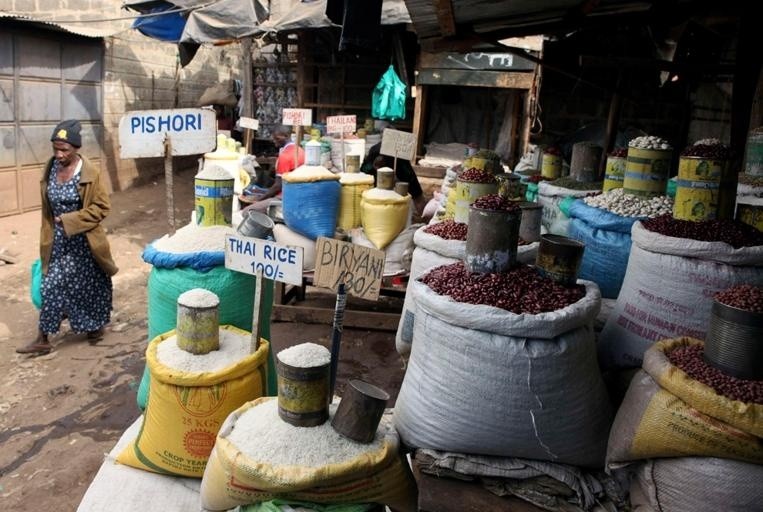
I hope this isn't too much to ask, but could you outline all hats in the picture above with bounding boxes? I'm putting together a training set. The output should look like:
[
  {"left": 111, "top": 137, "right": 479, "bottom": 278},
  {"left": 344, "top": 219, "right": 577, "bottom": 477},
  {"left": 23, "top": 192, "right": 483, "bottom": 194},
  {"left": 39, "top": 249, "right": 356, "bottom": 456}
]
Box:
[{"left": 51, "top": 120, "right": 81, "bottom": 148}]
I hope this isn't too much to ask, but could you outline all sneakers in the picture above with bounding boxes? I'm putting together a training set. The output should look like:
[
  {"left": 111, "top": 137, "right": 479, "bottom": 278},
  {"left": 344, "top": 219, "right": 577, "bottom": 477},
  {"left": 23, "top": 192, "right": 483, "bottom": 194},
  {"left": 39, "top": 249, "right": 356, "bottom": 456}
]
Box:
[
  {"left": 16, "top": 343, "right": 52, "bottom": 353},
  {"left": 87, "top": 326, "right": 104, "bottom": 339}
]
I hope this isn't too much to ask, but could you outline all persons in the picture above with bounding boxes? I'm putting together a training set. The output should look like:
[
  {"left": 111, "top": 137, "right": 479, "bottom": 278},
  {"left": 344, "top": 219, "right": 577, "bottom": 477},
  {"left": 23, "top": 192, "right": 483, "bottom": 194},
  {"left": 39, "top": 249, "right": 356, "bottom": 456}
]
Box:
[
  {"left": 360, "top": 123, "right": 425, "bottom": 214},
  {"left": 14, "top": 119, "right": 119, "bottom": 354},
  {"left": 238, "top": 123, "right": 304, "bottom": 217}
]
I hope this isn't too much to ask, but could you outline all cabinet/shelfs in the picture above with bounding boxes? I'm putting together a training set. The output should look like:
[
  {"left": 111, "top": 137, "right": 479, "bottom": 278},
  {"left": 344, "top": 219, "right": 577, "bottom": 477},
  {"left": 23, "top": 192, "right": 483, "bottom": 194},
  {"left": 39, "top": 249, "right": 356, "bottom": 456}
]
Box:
[
  {"left": 305, "top": 47, "right": 372, "bottom": 117},
  {"left": 242, "top": 34, "right": 306, "bottom": 162}
]
